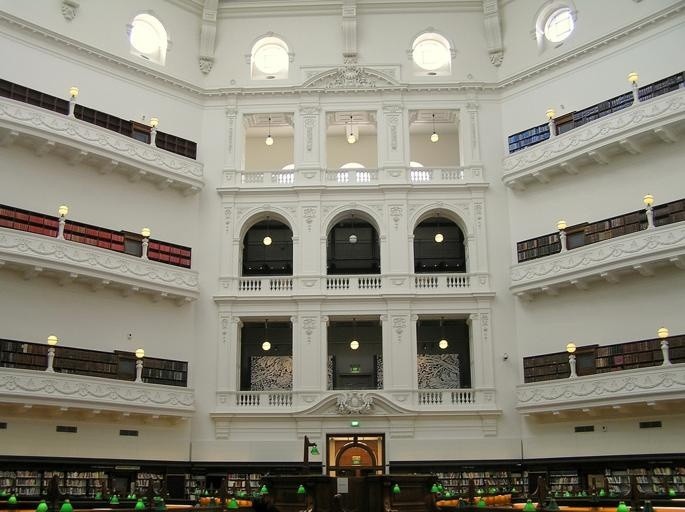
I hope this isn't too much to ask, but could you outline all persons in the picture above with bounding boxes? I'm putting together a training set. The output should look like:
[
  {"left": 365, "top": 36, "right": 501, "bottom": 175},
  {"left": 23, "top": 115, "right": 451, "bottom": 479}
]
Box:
[{"left": 45, "top": 474, "right": 65, "bottom": 511}]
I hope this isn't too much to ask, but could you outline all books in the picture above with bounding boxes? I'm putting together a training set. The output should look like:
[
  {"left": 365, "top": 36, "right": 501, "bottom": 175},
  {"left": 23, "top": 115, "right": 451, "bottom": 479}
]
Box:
[
  {"left": 1, "top": 469, "right": 269, "bottom": 497},
  {"left": 437, "top": 468, "right": 685, "bottom": 501}
]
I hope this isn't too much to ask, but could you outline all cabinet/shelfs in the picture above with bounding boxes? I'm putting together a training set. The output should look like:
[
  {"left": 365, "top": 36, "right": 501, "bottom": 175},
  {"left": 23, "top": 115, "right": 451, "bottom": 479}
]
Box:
[
  {"left": 0, "top": 79, "right": 197, "bottom": 161},
  {"left": 522, "top": 325, "right": 683, "bottom": 383},
  {"left": 507, "top": 70, "right": 684, "bottom": 157},
  {"left": 0, "top": 205, "right": 191, "bottom": 275},
  {"left": 0, "top": 337, "right": 187, "bottom": 387},
  {"left": 437, "top": 318, "right": 449, "bottom": 350},
  {"left": 0, "top": 454, "right": 685, "bottom": 512},
  {"left": 516, "top": 194, "right": 685, "bottom": 265}
]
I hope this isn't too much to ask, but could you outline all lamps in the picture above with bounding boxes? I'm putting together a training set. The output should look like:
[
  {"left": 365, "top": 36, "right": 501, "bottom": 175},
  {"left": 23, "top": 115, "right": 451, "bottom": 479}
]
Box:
[
  {"left": 265, "top": 115, "right": 274, "bottom": 146},
  {"left": 349, "top": 319, "right": 359, "bottom": 351},
  {"left": 262, "top": 320, "right": 270, "bottom": 351},
  {"left": 347, "top": 116, "right": 356, "bottom": 145},
  {"left": 263, "top": 217, "right": 272, "bottom": 246},
  {"left": 349, "top": 213, "right": 358, "bottom": 244},
  {"left": 303, "top": 435, "right": 319, "bottom": 463},
  {"left": 433, "top": 213, "right": 443, "bottom": 243},
  {"left": 429, "top": 113, "right": 439, "bottom": 142}
]
